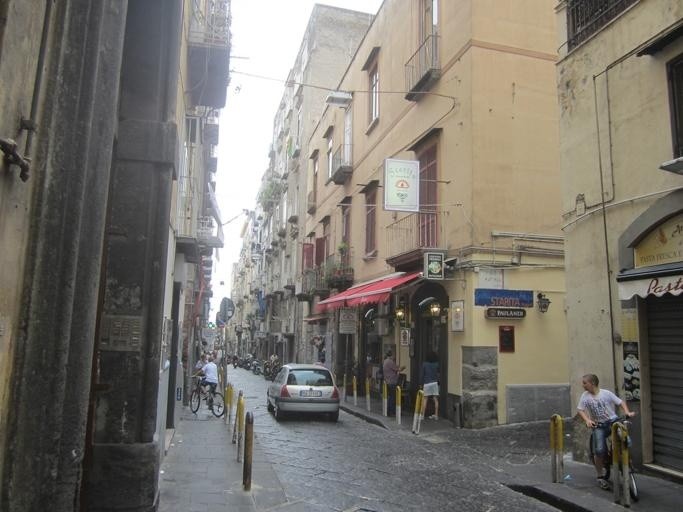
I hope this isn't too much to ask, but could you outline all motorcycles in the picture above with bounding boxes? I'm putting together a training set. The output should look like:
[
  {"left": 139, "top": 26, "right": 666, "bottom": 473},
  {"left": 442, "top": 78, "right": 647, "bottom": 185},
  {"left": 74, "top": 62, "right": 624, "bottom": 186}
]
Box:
[{"left": 227, "top": 356, "right": 282, "bottom": 380}]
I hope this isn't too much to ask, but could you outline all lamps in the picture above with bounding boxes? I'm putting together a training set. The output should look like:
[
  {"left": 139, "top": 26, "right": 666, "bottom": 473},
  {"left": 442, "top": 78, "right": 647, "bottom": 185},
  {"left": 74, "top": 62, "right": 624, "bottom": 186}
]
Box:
[{"left": 535, "top": 292, "right": 551, "bottom": 315}]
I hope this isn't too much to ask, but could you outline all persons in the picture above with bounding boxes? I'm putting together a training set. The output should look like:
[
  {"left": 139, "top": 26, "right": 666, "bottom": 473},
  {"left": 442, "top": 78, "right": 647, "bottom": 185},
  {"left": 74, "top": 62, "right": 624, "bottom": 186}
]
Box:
[
  {"left": 418, "top": 352, "right": 440, "bottom": 421},
  {"left": 228, "top": 355, "right": 241, "bottom": 370},
  {"left": 270, "top": 352, "right": 279, "bottom": 367},
  {"left": 196, "top": 355, "right": 208, "bottom": 374},
  {"left": 577, "top": 374, "right": 636, "bottom": 489},
  {"left": 382, "top": 351, "right": 406, "bottom": 414},
  {"left": 193, "top": 357, "right": 219, "bottom": 409}
]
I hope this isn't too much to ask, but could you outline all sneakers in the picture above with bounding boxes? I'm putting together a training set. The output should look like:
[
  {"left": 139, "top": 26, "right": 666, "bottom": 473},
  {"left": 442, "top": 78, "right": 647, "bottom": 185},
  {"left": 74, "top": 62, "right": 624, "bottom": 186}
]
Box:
[
  {"left": 202, "top": 394, "right": 208, "bottom": 400},
  {"left": 421, "top": 416, "right": 424, "bottom": 420},
  {"left": 596, "top": 476, "right": 609, "bottom": 490},
  {"left": 429, "top": 415, "right": 439, "bottom": 420}
]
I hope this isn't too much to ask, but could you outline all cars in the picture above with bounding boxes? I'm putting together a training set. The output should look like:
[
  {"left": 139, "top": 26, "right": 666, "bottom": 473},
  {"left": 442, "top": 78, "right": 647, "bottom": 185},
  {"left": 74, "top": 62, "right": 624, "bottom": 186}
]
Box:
[{"left": 267, "top": 364, "right": 339, "bottom": 421}]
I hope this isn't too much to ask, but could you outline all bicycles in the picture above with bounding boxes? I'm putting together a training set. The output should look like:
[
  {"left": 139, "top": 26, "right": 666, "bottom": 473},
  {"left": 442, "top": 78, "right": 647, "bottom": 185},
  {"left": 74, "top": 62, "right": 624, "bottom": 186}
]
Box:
[
  {"left": 190, "top": 376, "right": 225, "bottom": 417},
  {"left": 585, "top": 411, "right": 640, "bottom": 502}
]
